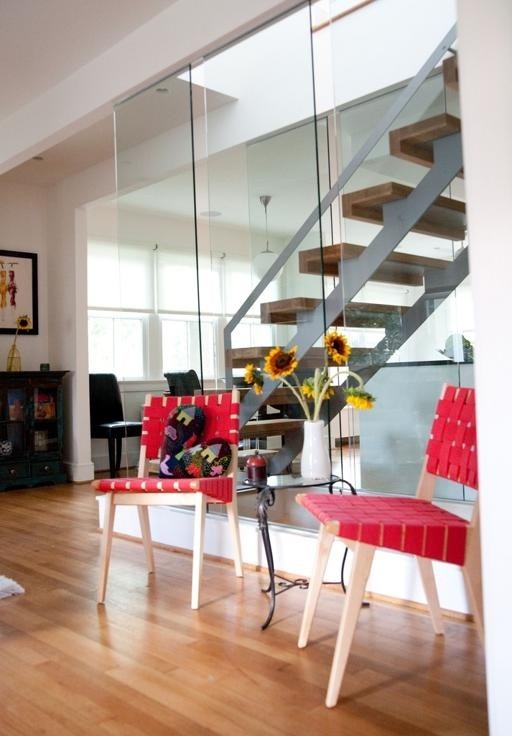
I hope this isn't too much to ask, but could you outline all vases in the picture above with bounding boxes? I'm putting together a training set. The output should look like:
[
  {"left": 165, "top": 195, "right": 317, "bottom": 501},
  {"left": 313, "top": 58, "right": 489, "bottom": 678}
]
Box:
[{"left": 6, "top": 344, "right": 23, "bottom": 373}]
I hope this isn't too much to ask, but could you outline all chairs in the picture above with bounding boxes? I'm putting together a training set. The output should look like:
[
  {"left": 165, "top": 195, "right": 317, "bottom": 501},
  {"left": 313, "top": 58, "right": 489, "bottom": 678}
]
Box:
[
  {"left": 91, "top": 388, "right": 245, "bottom": 611},
  {"left": 294, "top": 383, "right": 486, "bottom": 708}
]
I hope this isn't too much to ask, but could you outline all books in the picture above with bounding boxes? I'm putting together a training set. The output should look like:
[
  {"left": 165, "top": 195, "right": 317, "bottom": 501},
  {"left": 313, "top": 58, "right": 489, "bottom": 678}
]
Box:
[
  {"left": 33, "top": 388, "right": 56, "bottom": 421},
  {"left": 7, "top": 387, "right": 30, "bottom": 423}
]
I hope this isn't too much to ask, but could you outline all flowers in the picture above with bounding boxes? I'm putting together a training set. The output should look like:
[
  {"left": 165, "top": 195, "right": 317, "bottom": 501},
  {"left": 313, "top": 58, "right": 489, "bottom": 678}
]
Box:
[
  {"left": 246, "top": 333, "right": 379, "bottom": 420},
  {"left": 9, "top": 314, "right": 32, "bottom": 369}
]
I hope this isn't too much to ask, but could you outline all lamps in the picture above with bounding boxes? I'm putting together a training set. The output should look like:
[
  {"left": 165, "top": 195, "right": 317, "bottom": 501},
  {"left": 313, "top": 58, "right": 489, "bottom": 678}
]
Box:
[{"left": 251, "top": 195, "right": 283, "bottom": 285}]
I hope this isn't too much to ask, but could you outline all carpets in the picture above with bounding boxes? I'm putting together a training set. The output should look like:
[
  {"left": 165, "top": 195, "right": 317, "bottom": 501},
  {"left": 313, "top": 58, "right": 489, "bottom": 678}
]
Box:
[{"left": 0, "top": 575, "right": 25, "bottom": 600}]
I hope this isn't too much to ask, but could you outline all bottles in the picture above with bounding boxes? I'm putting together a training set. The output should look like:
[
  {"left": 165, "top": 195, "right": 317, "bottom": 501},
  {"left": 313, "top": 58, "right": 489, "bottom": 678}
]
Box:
[
  {"left": 6, "top": 345, "right": 21, "bottom": 371},
  {"left": 246, "top": 450, "right": 266, "bottom": 486}
]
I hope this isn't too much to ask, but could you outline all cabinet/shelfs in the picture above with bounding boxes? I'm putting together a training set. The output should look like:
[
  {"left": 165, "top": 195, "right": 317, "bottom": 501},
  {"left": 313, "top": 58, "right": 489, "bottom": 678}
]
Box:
[{"left": 0, "top": 371, "right": 73, "bottom": 492}]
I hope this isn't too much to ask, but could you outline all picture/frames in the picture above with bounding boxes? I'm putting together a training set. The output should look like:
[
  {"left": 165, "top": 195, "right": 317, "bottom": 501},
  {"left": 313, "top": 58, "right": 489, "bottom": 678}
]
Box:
[{"left": 0, "top": 250, "right": 39, "bottom": 334}]
